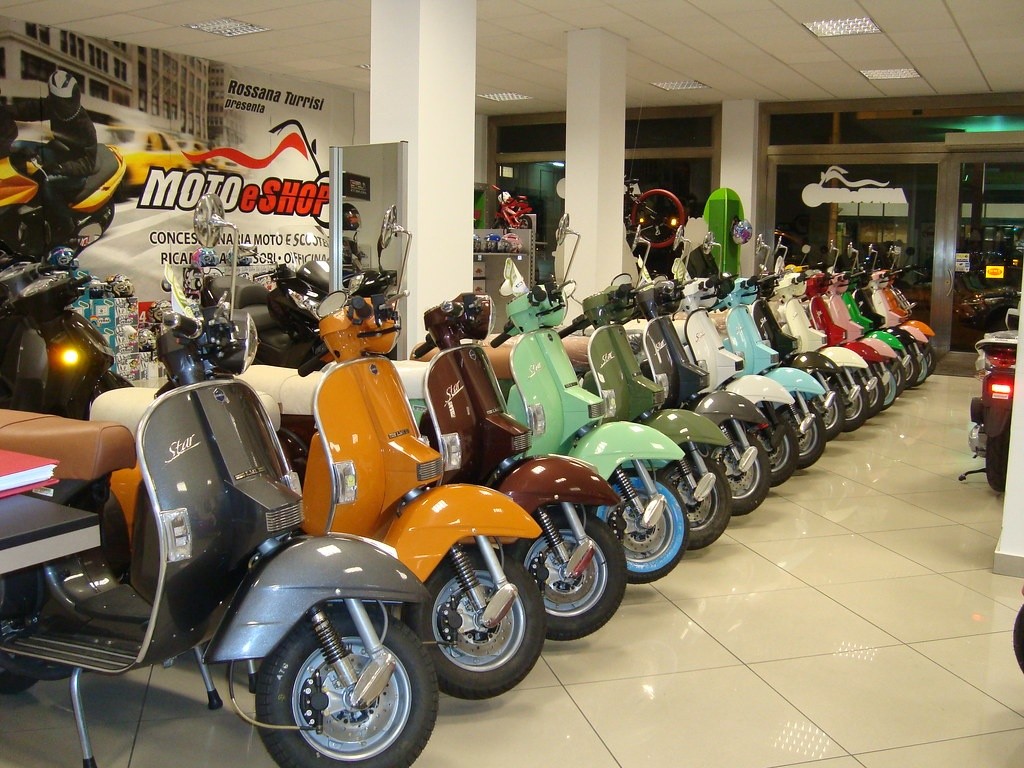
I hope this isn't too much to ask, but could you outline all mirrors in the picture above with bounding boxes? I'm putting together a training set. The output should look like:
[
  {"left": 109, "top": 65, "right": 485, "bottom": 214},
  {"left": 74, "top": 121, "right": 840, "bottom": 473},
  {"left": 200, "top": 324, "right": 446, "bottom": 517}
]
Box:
[{"left": 329, "top": 140, "right": 409, "bottom": 363}]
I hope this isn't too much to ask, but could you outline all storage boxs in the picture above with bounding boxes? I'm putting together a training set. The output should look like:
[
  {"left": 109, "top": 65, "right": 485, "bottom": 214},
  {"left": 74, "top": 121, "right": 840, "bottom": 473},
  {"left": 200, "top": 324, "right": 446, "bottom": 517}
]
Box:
[
  {"left": 474, "top": 261, "right": 486, "bottom": 293},
  {"left": 68, "top": 269, "right": 164, "bottom": 381}
]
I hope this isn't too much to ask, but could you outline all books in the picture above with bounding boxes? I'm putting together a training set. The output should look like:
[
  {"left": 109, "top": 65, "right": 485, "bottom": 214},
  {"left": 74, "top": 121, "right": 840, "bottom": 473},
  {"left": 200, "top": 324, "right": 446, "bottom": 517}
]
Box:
[{"left": 0, "top": 448, "right": 62, "bottom": 498}]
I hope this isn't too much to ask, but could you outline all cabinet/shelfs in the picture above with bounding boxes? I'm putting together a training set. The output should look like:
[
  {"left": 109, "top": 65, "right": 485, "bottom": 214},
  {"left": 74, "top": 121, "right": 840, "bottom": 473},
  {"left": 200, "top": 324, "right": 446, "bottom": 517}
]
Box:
[{"left": 474, "top": 228, "right": 533, "bottom": 335}]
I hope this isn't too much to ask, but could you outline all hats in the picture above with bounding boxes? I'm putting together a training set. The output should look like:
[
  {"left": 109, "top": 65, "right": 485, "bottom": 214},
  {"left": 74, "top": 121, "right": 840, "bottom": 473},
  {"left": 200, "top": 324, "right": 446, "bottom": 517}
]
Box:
[{"left": 483, "top": 233, "right": 501, "bottom": 252}]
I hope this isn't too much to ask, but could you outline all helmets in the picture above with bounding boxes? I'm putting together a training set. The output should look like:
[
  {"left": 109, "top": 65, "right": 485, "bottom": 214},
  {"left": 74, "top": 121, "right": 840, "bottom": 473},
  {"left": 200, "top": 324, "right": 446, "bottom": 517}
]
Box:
[
  {"left": 498, "top": 233, "right": 523, "bottom": 252},
  {"left": 191, "top": 248, "right": 219, "bottom": 267},
  {"left": 148, "top": 299, "right": 172, "bottom": 323},
  {"left": 473, "top": 232, "right": 482, "bottom": 251},
  {"left": 103, "top": 274, "right": 134, "bottom": 298},
  {"left": 48, "top": 72, "right": 80, "bottom": 120},
  {"left": 732, "top": 219, "right": 753, "bottom": 245},
  {"left": 137, "top": 327, "right": 156, "bottom": 352},
  {"left": 46, "top": 246, "right": 80, "bottom": 269},
  {"left": 343, "top": 202, "right": 362, "bottom": 230}
]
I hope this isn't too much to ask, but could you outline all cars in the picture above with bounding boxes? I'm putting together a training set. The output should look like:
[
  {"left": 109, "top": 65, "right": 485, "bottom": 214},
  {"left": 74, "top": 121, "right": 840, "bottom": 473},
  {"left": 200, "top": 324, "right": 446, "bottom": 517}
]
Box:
[{"left": 99, "top": 124, "right": 240, "bottom": 197}]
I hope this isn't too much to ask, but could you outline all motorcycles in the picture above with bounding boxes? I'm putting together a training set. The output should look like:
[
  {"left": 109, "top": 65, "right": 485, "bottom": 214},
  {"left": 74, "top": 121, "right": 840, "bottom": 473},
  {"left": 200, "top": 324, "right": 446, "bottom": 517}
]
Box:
[{"left": 1, "top": 104, "right": 127, "bottom": 261}]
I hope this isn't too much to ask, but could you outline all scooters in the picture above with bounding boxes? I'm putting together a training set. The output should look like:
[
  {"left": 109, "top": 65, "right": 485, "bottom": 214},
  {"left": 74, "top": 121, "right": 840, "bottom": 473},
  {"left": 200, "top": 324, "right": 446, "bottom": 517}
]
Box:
[
  {"left": 408, "top": 273, "right": 732, "bottom": 550},
  {"left": 234, "top": 290, "right": 629, "bottom": 642},
  {"left": 1, "top": 192, "right": 439, "bottom": 767},
  {"left": 390, "top": 213, "right": 691, "bottom": 585},
  {"left": 957, "top": 289, "right": 1021, "bottom": 491},
  {"left": 87, "top": 204, "right": 548, "bottom": 702},
  {"left": 573, "top": 232, "right": 937, "bottom": 469},
  {"left": 479, "top": 224, "right": 774, "bottom": 518},
  {"left": 563, "top": 223, "right": 800, "bottom": 487},
  {"left": 1, "top": 222, "right": 396, "bottom": 421}
]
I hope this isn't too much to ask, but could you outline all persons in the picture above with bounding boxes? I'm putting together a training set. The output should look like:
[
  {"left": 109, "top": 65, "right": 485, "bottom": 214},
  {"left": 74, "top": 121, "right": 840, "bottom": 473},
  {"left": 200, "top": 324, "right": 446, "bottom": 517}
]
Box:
[{"left": 0, "top": 67, "right": 98, "bottom": 261}]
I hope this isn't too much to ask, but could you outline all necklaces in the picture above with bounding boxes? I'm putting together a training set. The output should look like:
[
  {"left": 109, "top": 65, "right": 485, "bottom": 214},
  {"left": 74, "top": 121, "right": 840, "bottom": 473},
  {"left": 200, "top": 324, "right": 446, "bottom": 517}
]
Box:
[{"left": 55, "top": 107, "right": 81, "bottom": 121}]
